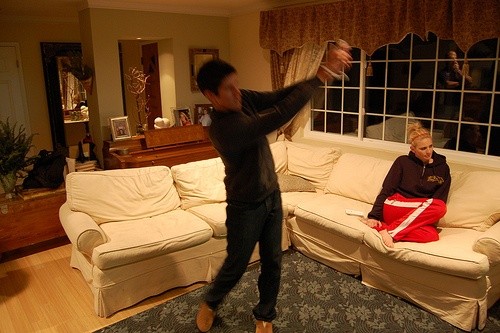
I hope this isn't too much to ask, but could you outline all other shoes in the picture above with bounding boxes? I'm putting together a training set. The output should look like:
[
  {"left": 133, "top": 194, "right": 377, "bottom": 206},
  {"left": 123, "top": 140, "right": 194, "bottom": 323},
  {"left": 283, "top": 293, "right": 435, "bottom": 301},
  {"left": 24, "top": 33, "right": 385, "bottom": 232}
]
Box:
[
  {"left": 255, "top": 320, "right": 272, "bottom": 333},
  {"left": 192, "top": 298, "right": 218, "bottom": 333}
]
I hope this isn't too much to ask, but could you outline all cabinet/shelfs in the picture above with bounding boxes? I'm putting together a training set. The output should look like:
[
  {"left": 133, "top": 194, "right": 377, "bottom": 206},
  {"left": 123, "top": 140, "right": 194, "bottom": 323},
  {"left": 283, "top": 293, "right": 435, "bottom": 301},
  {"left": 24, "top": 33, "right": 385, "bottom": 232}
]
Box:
[
  {"left": 0, "top": 185, "right": 66, "bottom": 253},
  {"left": 103, "top": 125, "right": 221, "bottom": 170}
]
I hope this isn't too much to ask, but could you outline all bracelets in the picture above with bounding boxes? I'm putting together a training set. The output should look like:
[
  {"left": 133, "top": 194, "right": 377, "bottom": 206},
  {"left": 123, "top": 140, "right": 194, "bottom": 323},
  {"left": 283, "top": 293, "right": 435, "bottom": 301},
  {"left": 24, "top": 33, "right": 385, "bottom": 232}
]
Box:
[{"left": 319, "top": 62, "right": 349, "bottom": 80}]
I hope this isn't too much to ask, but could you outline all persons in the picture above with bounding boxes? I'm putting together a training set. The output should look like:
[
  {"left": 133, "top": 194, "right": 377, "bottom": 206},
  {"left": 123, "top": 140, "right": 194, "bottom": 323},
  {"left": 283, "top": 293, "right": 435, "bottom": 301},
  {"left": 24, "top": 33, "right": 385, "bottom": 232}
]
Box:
[
  {"left": 360, "top": 121, "right": 452, "bottom": 249},
  {"left": 197, "top": 38, "right": 352, "bottom": 333},
  {"left": 200, "top": 110, "right": 211, "bottom": 126},
  {"left": 179, "top": 113, "right": 191, "bottom": 125}
]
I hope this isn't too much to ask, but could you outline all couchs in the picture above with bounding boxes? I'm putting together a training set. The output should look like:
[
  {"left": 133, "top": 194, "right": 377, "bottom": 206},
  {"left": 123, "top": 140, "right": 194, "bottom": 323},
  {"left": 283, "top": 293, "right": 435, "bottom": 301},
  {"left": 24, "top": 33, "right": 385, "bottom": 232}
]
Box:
[
  {"left": 344, "top": 112, "right": 448, "bottom": 148},
  {"left": 58, "top": 140, "right": 500, "bottom": 333}
]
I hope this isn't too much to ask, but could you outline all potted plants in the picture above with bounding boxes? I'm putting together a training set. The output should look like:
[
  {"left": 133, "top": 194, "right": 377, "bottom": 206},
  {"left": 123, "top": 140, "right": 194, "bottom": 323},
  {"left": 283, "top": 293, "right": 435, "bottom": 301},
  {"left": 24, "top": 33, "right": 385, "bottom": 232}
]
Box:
[{"left": 0, "top": 117, "right": 35, "bottom": 199}]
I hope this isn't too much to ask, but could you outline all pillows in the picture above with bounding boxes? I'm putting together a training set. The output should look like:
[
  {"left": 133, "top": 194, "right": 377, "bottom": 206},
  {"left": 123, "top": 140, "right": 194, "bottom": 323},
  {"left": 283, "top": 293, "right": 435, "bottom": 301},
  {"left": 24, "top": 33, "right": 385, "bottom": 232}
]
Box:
[{"left": 277, "top": 174, "right": 316, "bottom": 192}]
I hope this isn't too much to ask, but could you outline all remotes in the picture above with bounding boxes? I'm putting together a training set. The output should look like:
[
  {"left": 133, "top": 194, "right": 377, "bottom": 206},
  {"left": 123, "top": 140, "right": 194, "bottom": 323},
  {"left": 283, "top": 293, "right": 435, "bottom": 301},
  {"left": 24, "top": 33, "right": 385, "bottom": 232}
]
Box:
[{"left": 345, "top": 210, "right": 363, "bottom": 216}]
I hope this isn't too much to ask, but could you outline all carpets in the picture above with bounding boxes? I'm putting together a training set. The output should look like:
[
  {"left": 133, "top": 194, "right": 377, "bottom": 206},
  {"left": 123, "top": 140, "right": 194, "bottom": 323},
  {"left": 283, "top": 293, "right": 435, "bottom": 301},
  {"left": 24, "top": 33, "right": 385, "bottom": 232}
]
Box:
[{"left": 92, "top": 248, "right": 500, "bottom": 333}]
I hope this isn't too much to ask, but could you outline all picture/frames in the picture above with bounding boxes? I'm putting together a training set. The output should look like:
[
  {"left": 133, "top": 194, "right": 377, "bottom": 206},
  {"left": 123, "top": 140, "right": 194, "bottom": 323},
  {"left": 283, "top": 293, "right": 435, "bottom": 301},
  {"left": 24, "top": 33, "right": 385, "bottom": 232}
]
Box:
[
  {"left": 194, "top": 104, "right": 213, "bottom": 125},
  {"left": 110, "top": 116, "right": 131, "bottom": 139},
  {"left": 173, "top": 107, "right": 193, "bottom": 126},
  {"left": 189, "top": 48, "right": 219, "bottom": 93}
]
